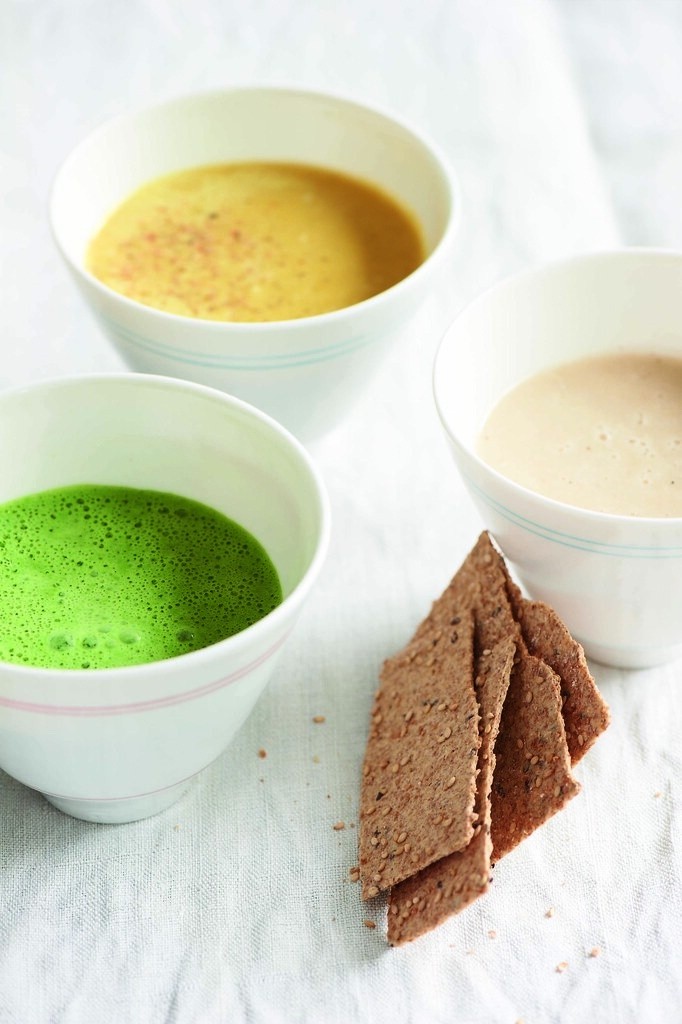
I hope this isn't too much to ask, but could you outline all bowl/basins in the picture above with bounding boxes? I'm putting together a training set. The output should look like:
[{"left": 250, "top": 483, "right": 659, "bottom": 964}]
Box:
[
  {"left": 48, "top": 86, "right": 455, "bottom": 443},
  {"left": 433, "top": 247, "right": 682, "bottom": 670},
  {"left": 0, "top": 374, "right": 330, "bottom": 824}
]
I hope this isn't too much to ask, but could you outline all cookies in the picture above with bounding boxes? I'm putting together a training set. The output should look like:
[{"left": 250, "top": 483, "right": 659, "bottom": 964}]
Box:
[{"left": 359, "top": 533, "right": 611, "bottom": 944}]
